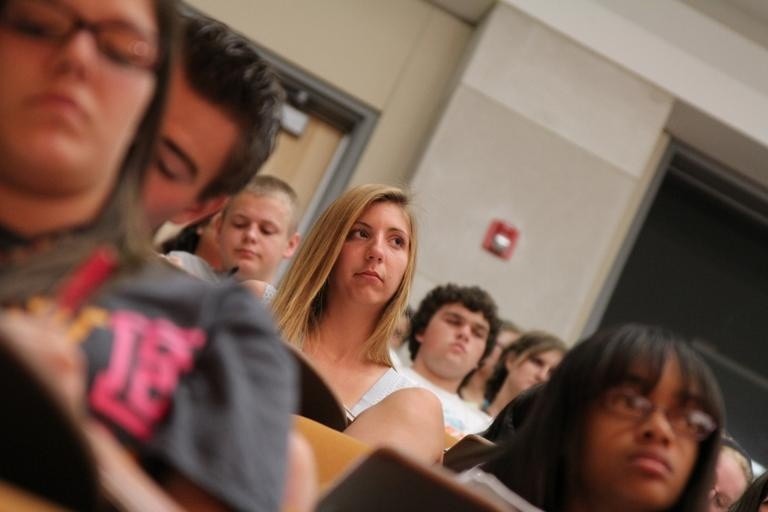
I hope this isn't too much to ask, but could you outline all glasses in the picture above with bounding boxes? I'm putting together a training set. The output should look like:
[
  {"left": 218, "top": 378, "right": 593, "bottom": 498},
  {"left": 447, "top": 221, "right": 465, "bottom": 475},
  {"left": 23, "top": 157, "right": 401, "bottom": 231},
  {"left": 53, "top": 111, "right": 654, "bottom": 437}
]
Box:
[
  {"left": 602, "top": 383, "right": 718, "bottom": 444},
  {"left": 1, "top": 2, "right": 163, "bottom": 72}
]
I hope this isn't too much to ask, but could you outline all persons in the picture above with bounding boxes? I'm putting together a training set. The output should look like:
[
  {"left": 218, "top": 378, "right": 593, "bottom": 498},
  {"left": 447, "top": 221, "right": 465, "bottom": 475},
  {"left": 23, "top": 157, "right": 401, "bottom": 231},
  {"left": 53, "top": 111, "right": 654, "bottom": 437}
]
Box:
[
  {"left": 688, "top": 432, "right": 757, "bottom": 511},
  {"left": 480, "top": 330, "right": 575, "bottom": 418},
  {"left": 209, "top": 174, "right": 300, "bottom": 291},
  {"left": 456, "top": 319, "right": 522, "bottom": 405},
  {"left": 121, "top": 10, "right": 285, "bottom": 270},
  {"left": 504, "top": 314, "right": 729, "bottom": 512},
  {"left": 725, "top": 466, "right": 768, "bottom": 512},
  {"left": 226, "top": 179, "right": 454, "bottom": 473},
  {"left": 405, "top": 280, "right": 502, "bottom": 398},
  {"left": 0, "top": 0, "right": 316, "bottom": 511},
  {"left": 155, "top": 203, "right": 231, "bottom": 279},
  {"left": 384, "top": 298, "right": 417, "bottom": 349}
]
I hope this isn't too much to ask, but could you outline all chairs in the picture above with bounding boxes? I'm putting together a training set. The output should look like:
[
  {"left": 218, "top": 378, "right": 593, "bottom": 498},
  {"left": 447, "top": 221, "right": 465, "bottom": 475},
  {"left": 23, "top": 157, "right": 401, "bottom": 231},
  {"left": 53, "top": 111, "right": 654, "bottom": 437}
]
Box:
[{"left": 287, "top": 414, "right": 373, "bottom": 501}]
